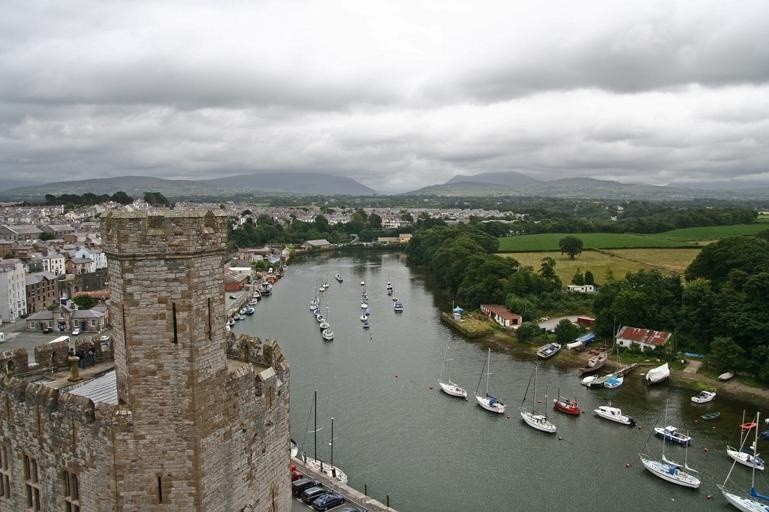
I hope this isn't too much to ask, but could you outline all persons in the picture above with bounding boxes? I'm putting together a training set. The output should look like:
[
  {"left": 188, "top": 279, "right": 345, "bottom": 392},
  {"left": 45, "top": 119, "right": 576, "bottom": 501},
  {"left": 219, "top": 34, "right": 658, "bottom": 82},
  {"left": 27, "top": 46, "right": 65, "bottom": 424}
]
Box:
[{"left": 66, "top": 345, "right": 97, "bottom": 369}]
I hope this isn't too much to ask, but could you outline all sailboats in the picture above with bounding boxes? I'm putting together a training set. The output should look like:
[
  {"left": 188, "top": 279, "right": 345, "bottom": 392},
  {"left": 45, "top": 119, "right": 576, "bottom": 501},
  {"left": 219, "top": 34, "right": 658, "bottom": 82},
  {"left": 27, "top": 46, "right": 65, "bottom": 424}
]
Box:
[
  {"left": 638, "top": 398, "right": 701, "bottom": 489},
  {"left": 472, "top": 347, "right": 506, "bottom": 414},
  {"left": 715, "top": 417, "right": 769, "bottom": 512},
  {"left": 748, "top": 411, "right": 769, "bottom": 506},
  {"left": 517, "top": 366, "right": 558, "bottom": 434},
  {"left": 302, "top": 390, "right": 349, "bottom": 485}
]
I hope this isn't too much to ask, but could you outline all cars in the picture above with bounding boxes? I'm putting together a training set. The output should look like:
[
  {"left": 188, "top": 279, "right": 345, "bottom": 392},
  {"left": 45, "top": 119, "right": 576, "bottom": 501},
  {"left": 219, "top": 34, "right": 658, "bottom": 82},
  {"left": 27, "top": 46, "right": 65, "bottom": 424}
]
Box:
[
  {"left": 291, "top": 471, "right": 362, "bottom": 512},
  {"left": 71, "top": 326, "right": 81, "bottom": 336},
  {"left": 99, "top": 335, "right": 109, "bottom": 341}
]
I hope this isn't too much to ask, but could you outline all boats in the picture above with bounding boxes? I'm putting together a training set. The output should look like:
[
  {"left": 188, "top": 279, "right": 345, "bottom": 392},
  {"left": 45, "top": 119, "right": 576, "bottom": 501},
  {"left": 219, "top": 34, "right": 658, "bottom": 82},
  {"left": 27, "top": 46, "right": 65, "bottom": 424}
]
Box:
[
  {"left": 726, "top": 445, "right": 766, "bottom": 471},
  {"left": 644, "top": 362, "right": 672, "bottom": 387},
  {"left": 536, "top": 342, "right": 562, "bottom": 358},
  {"left": 552, "top": 383, "right": 581, "bottom": 416},
  {"left": 579, "top": 323, "right": 638, "bottom": 388},
  {"left": 356, "top": 276, "right": 370, "bottom": 331},
  {"left": 739, "top": 422, "right": 757, "bottom": 429},
  {"left": 593, "top": 401, "right": 637, "bottom": 427},
  {"left": 579, "top": 350, "right": 608, "bottom": 374},
  {"left": 717, "top": 368, "right": 737, "bottom": 382},
  {"left": 603, "top": 357, "right": 625, "bottom": 389},
  {"left": 690, "top": 388, "right": 718, "bottom": 404},
  {"left": 436, "top": 376, "right": 469, "bottom": 401},
  {"left": 383, "top": 281, "right": 403, "bottom": 312},
  {"left": 700, "top": 411, "right": 721, "bottom": 421},
  {"left": 225, "top": 264, "right": 289, "bottom": 335},
  {"left": 653, "top": 425, "right": 691, "bottom": 445},
  {"left": 308, "top": 272, "right": 344, "bottom": 344}
]
